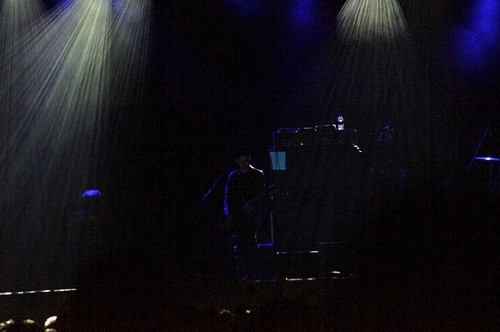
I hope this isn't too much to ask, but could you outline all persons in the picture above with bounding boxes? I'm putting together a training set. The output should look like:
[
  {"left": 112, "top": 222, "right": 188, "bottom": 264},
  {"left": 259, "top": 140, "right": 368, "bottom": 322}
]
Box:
[{"left": 224, "top": 148, "right": 265, "bottom": 242}]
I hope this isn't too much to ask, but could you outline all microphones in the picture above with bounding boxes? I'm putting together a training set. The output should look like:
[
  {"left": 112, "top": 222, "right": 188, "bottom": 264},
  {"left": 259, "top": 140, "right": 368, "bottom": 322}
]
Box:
[{"left": 221, "top": 168, "right": 229, "bottom": 178}]
